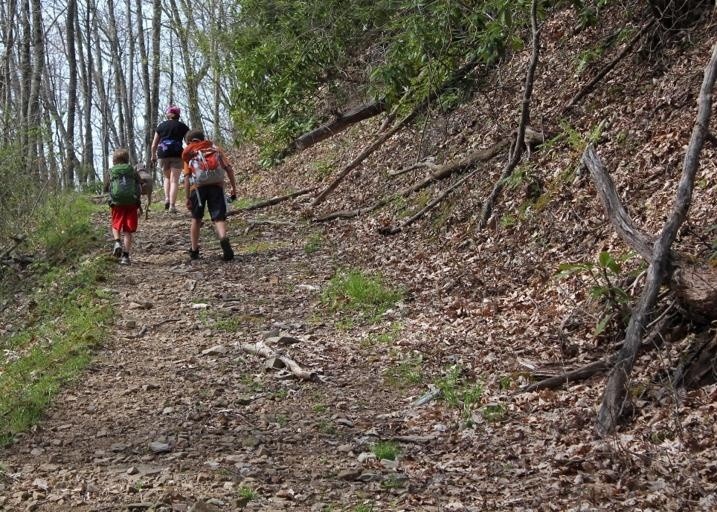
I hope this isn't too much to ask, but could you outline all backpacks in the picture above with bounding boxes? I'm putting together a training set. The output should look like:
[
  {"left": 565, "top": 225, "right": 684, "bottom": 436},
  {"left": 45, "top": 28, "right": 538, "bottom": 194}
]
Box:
[
  {"left": 109, "top": 164, "right": 137, "bottom": 205},
  {"left": 182, "top": 141, "right": 225, "bottom": 185}
]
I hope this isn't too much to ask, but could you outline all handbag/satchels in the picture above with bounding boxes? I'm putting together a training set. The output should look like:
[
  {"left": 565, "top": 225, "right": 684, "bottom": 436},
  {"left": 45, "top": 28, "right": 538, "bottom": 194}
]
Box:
[{"left": 160, "top": 141, "right": 180, "bottom": 153}]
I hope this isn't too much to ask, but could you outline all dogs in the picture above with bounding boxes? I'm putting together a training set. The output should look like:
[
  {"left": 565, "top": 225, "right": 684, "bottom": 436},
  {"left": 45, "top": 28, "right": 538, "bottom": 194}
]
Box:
[{"left": 134, "top": 163, "right": 154, "bottom": 221}]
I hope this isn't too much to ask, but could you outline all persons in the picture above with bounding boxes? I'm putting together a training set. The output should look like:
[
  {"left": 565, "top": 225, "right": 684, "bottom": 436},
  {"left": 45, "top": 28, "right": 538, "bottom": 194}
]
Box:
[
  {"left": 148, "top": 106, "right": 192, "bottom": 213},
  {"left": 100, "top": 147, "right": 143, "bottom": 266},
  {"left": 180, "top": 128, "right": 237, "bottom": 262}
]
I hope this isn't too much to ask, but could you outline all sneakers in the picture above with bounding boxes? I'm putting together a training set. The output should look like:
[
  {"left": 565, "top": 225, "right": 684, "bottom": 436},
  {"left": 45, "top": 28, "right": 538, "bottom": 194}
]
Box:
[
  {"left": 188, "top": 248, "right": 198, "bottom": 258},
  {"left": 220, "top": 236, "right": 234, "bottom": 261},
  {"left": 120, "top": 258, "right": 131, "bottom": 266},
  {"left": 113, "top": 242, "right": 122, "bottom": 256}
]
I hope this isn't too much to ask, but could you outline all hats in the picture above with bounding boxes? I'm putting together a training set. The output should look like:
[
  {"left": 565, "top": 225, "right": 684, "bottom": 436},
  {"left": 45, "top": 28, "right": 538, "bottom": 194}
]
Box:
[{"left": 166, "top": 107, "right": 181, "bottom": 114}]
